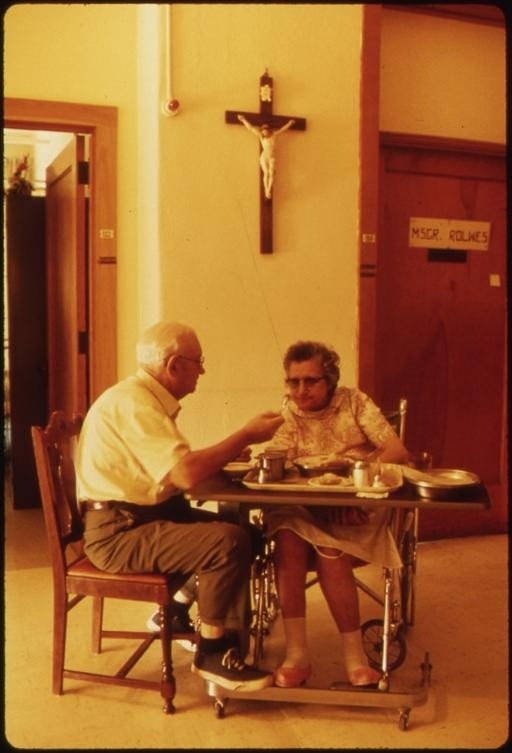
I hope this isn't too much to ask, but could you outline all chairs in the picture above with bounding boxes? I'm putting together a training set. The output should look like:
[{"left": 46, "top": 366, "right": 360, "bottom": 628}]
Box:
[{"left": 31, "top": 411, "right": 201, "bottom": 716}]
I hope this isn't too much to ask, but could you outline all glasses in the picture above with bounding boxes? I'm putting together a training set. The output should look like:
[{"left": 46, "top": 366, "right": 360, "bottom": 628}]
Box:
[
  {"left": 164, "top": 352, "right": 205, "bottom": 367},
  {"left": 285, "top": 375, "right": 326, "bottom": 384}
]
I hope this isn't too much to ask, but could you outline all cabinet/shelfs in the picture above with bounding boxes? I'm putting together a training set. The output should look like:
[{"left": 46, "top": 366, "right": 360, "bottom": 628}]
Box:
[{"left": 2, "top": 195, "right": 46, "bottom": 509}]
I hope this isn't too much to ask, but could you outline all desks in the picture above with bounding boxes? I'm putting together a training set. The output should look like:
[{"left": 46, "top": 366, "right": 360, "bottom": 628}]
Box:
[{"left": 185, "top": 474, "right": 490, "bottom": 729}]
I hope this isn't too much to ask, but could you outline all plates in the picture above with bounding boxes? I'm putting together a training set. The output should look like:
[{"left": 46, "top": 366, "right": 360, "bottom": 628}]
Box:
[
  {"left": 306, "top": 475, "right": 402, "bottom": 492},
  {"left": 291, "top": 455, "right": 354, "bottom": 477}
]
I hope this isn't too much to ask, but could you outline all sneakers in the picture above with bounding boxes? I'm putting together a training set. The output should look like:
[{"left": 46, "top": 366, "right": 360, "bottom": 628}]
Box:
[
  {"left": 349, "top": 667, "right": 380, "bottom": 687},
  {"left": 147, "top": 611, "right": 198, "bottom": 653},
  {"left": 276, "top": 663, "right": 311, "bottom": 688},
  {"left": 190, "top": 647, "right": 273, "bottom": 692}
]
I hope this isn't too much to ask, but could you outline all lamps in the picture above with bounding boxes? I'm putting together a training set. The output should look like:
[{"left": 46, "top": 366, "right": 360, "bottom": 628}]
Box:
[{"left": 161, "top": 97, "right": 181, "bottom": 116}]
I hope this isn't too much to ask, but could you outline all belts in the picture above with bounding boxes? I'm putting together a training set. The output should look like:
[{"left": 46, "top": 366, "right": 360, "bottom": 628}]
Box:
[{"left": 81, "top": 501, "right": 124, "bottom": 511}]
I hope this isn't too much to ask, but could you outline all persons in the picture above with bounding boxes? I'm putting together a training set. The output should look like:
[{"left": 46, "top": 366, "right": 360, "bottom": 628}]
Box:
[
  {"left": 74, "top": 320, "right": 285, "bottom": 694},
  {"left": 237, "top": 113, "right": 295, "bottom": 200},
  {"left": 268, "top": 338, "right": 411, "bottom": 689}
]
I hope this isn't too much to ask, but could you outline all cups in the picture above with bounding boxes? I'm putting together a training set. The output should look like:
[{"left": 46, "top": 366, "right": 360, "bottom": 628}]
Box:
[{"left": 263, "top": 445, "right": 288, "bottom": 480}]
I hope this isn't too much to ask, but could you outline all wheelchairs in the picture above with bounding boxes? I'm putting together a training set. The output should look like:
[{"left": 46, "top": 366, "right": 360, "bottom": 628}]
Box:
[{"left": 248, "top": 397, "right": 417, "bottom": 672}]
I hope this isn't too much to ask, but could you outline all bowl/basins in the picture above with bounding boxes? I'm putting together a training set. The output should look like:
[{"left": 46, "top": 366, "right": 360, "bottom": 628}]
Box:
[
  {"left": 224, "top": 461, "right": 252, "bottom": 481},
  {"left": 408, "top": 468, "right": 480, "bottom": 499}
]
